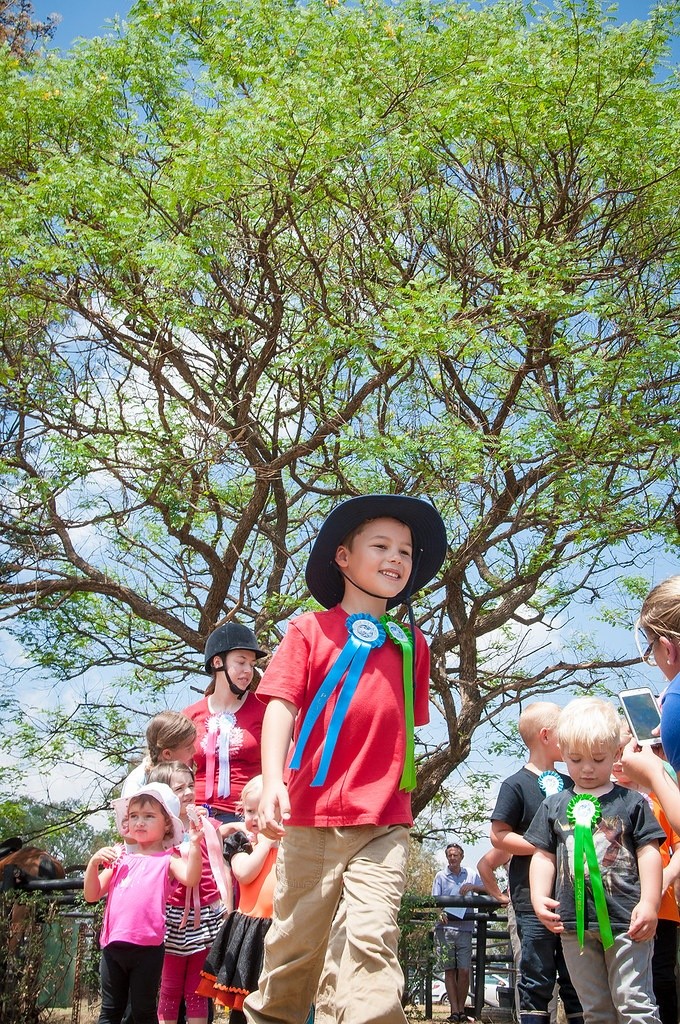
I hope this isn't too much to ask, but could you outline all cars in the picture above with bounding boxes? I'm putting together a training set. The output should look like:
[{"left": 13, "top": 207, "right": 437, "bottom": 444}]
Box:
[{"left": 406, "top": 962, "right": 508, "bottom": 1009}]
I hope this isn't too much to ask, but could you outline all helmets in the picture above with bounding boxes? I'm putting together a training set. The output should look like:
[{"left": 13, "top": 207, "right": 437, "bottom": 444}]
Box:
[{"left": 204, "top": 622, "right": 268, "bottom": 674}]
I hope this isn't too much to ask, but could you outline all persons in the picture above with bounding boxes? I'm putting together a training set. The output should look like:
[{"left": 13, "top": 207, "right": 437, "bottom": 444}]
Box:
[
  {"left": 432, "top": 576, "right": 680, "bottom": 1024},
  {"left": 83, "top": 494, "right": 447, "bottom": 1024}
]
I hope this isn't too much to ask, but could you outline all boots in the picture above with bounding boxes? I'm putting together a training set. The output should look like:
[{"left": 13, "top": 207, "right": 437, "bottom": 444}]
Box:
[
  {"left": 520, "top": 1009, "right": 551, "bottom": 1024},
  {"left": 567, "top": 1012, "right": 584, "bottom": 1024}
]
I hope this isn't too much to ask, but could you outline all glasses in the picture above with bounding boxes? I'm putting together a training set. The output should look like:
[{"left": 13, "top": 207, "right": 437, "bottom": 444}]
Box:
[{"left": 643, "top": 632, "right": 659, "bottom": 666}]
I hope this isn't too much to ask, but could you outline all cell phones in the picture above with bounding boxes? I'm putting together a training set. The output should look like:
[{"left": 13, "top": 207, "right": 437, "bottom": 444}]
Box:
[{"left": 618, "top": 687, "right": 663, "bottom": 747}]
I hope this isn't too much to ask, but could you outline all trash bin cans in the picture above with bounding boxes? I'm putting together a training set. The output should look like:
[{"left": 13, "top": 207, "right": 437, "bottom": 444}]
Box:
[
  {"left": 36, "top": 920, "right": 79, "bottom": 1008},
  {"left": 497, "top": 986, "right": 515, "bottom": 1007}
]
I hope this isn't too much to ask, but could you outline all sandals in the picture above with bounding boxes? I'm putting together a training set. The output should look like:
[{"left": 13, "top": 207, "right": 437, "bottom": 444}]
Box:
[
  {"left": 447, "top": 1012, "right": 459, "bottom": 1024},
  {"left": 459, "top": 1013, "right": 470, "bottom": 1024}
]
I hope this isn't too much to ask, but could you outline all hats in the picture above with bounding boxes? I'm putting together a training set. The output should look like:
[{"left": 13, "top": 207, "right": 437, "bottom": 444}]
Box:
[
  {"left": 305, "top": 494, "right": 447, "bottom": 611},
  {"left": 112, "top": 782, "right": 185, "bottom": 849}
]
